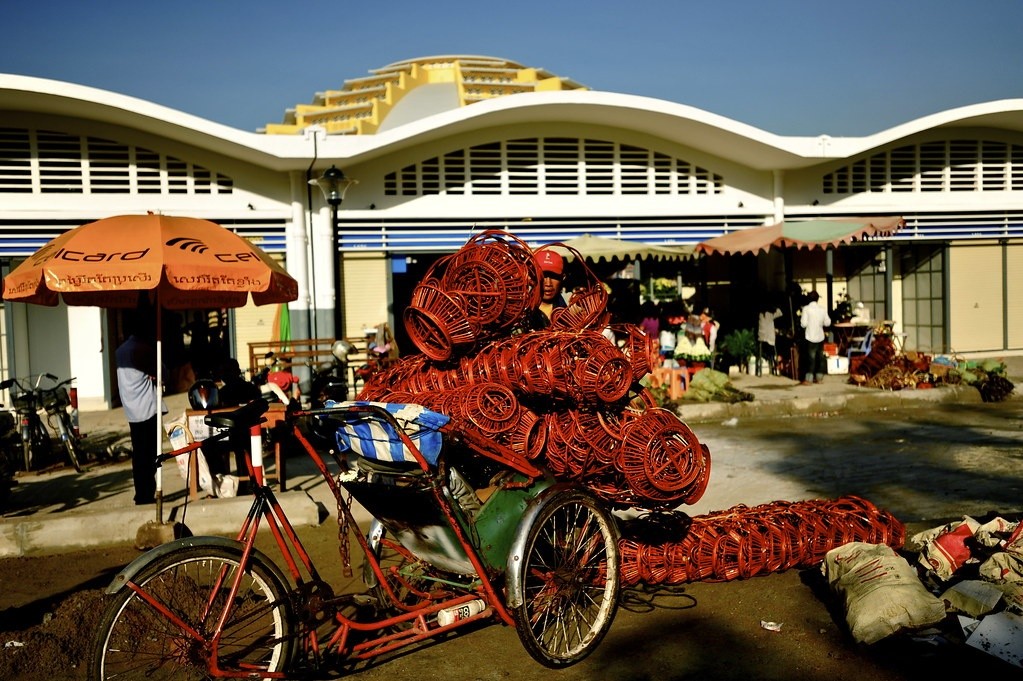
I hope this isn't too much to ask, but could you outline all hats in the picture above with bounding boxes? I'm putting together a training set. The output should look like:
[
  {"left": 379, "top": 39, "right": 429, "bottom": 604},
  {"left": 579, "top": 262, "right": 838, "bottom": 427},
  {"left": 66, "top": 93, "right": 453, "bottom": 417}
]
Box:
[{"left": 533, "top": 249, "right": 564, "bottom": 275}]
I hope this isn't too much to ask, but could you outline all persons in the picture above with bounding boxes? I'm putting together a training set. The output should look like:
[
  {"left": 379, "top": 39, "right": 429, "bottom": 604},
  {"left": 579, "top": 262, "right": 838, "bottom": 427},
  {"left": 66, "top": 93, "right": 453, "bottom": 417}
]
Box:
[
  {"left": 799, "top": 291, "right": 835, "bottom": 382},
  {"left": 114, "top": 316, "right": 168, "bottom": 504},
  {"left": 205, "top": 358, "right": 262, "bottom": 475},
  {"left": 512, "top": 251, "right": 571, "bottom": 337},
  {"left": 638, "top": 300, "right": 662, "bottom": 348}
]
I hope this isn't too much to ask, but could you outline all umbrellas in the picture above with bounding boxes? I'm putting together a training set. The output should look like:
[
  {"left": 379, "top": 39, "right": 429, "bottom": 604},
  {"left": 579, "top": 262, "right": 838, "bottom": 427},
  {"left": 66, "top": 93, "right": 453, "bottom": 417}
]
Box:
[
  {"left": 2, "top": 215, "right": 299, "bottom": 526},
  {"left": 532, "top": 229, "right": 699, "bottom": 271},
  {"left": 696, "top": 212, "right": 906, "bottom": 343}
]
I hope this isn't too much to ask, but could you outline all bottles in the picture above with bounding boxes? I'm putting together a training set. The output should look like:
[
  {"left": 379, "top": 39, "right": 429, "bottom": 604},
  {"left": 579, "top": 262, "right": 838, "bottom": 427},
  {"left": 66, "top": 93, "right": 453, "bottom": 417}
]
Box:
[{"left": 437, "top": 599, "right": 486, "bottom": 627}]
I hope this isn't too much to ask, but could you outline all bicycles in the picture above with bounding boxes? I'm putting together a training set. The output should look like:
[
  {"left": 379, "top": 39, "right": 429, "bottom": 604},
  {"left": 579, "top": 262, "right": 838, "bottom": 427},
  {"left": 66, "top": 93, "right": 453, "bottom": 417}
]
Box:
[
  {"left": 0, "top": 373, "right": 59, "bottom": 474},
  {"left": 33, "top": 377, "right": 84, "bottom": 474}
]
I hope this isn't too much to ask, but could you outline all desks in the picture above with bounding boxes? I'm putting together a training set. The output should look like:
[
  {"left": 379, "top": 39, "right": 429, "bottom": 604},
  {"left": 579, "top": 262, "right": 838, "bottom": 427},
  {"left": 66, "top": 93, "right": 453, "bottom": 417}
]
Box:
[{"left": 186, "top": 403, "right": 289, "bottom": 500}]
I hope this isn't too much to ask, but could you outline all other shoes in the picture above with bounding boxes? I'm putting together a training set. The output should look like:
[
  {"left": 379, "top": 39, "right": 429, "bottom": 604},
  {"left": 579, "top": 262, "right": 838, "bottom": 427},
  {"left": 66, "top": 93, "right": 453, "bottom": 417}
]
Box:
[
  {"left": 132, "top": 496, "right": 156, "bottom": 505},
  {"left": 816, "top": 379, "right": 823, "bottom": 383},
  {"left": 802, "top": 380, "right": 810, "bottom": 385}
]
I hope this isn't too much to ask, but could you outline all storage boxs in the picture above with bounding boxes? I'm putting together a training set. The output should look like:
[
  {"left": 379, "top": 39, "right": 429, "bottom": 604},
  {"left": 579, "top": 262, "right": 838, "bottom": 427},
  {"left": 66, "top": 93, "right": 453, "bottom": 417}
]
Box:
[{"left": 823, "top": 342, "right": 850, "bottom": 374}]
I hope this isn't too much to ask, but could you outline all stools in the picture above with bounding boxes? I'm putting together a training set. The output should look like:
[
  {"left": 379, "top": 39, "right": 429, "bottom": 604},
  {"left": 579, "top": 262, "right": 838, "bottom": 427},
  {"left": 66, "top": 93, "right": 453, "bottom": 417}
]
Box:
[
  {"left": 650, "top": 360, "right": 704, "bottom": 400},
  {"left": 755, "top": 339, "right": 777, "bottom": 378}
]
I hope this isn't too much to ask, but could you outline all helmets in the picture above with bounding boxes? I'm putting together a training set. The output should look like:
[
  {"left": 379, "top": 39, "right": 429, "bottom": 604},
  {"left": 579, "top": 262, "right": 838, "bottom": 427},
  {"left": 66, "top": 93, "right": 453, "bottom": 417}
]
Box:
[
  {"left": 331, "top": 340, "right": 353, "bottom": 363},
  {"left": 188, "top": 379, "right": 222, "bottom": 410}
]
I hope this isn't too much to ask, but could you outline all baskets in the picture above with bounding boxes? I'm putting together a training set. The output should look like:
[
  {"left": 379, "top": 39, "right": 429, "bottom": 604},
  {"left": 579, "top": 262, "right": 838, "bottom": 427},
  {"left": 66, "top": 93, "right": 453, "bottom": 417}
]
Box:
[
  {"left": 11, "top": 390, "right": 42, "bottom": 412},
  {"left": 358, "top": 231, "right": 712, "bottom": 512},
  {"left": 575, "top": 496, "right": 907, "bottom": 587},
  {"left": 39, "top": 387, "right": 71, "bottom": 414}
]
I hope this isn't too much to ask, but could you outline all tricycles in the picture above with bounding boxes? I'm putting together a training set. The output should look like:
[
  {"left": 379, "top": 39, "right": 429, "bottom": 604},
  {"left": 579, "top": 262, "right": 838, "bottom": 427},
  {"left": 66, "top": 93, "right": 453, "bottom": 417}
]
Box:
[{"left": 80, "top": 383, "right": 624, "bottom": 681}]
